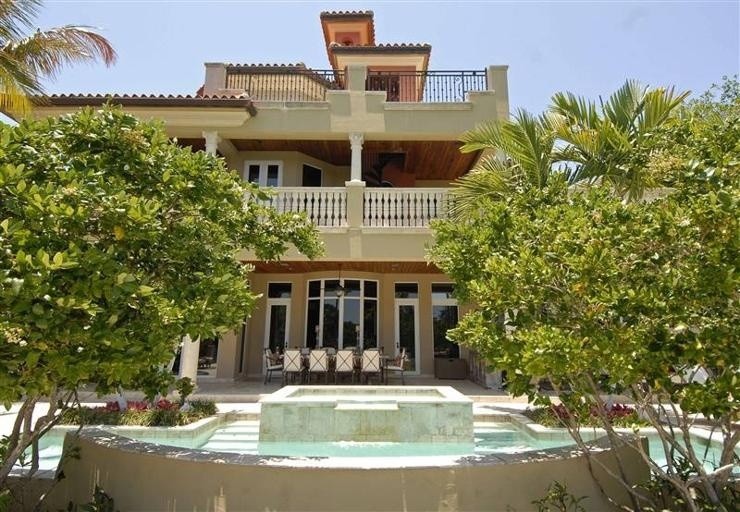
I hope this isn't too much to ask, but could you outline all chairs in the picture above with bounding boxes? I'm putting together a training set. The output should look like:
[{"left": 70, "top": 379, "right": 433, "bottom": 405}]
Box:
[{"left": 264, "top": 346, "right": 407, "bottom": 387}]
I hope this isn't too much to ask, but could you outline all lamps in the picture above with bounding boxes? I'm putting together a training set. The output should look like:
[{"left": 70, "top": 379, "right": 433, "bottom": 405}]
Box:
[{"left": 334, "top": 263, "right": 346, "bottom": 296}]
